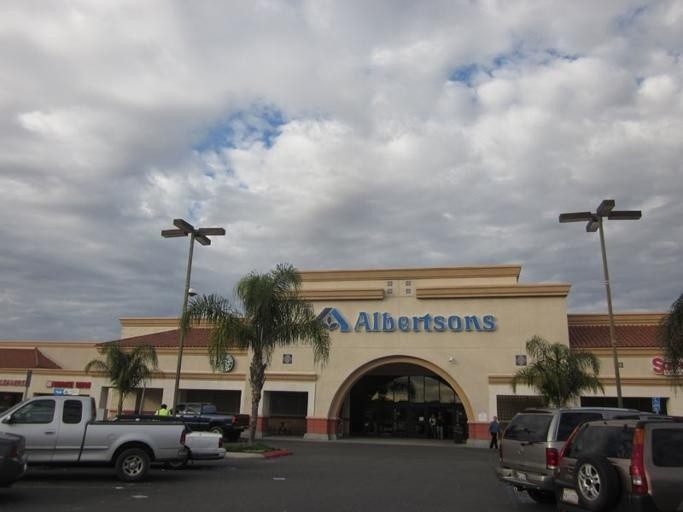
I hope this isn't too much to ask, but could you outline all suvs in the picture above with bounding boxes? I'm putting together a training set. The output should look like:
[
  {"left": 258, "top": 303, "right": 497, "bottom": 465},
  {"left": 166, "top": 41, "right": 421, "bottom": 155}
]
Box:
[{"left": 492, "top": 407, "right": 683, "bottom": 512}]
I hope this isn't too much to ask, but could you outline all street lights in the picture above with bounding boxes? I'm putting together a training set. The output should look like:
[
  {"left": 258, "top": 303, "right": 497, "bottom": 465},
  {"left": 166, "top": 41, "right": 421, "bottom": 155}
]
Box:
[
  {"left": 160, "top": 218, "right": 226, "bottom": 420},
  {"left": 559, "top": 199, "right": 642, "bottom": 408}
]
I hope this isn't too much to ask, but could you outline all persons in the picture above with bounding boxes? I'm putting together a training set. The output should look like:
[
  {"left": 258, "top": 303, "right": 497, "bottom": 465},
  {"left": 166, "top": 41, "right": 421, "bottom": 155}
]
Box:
[
  {"left": 487, "top": 416, "right": 501, "bottom": 453},
  {"left": 436, "top": 411, "right": 445, "bottom": 440},
  {"left": 428, "top": 413, "right": 437, "bottom": 439},
  {"left": 154, "top": 404, "right": 171, "bottom": 416}
]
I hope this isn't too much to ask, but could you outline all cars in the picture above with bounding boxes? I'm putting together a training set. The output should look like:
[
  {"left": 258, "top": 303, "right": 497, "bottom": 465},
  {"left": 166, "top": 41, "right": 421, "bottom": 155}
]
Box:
[
  {"left": 109, "top": 415, "right": 225, "bottom": 469},
  {"left": 0, "top": 431, "right": 27, "bottom": 489}
]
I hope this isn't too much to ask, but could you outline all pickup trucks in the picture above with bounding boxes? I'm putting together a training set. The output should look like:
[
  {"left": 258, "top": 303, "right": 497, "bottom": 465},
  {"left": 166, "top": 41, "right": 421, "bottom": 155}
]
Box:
[
  {"left": 169, "top": 402, "right": 250, "bottom": 441},
  {"left": 0, "top": 396, "right": 189, "bottom": 483}
]
